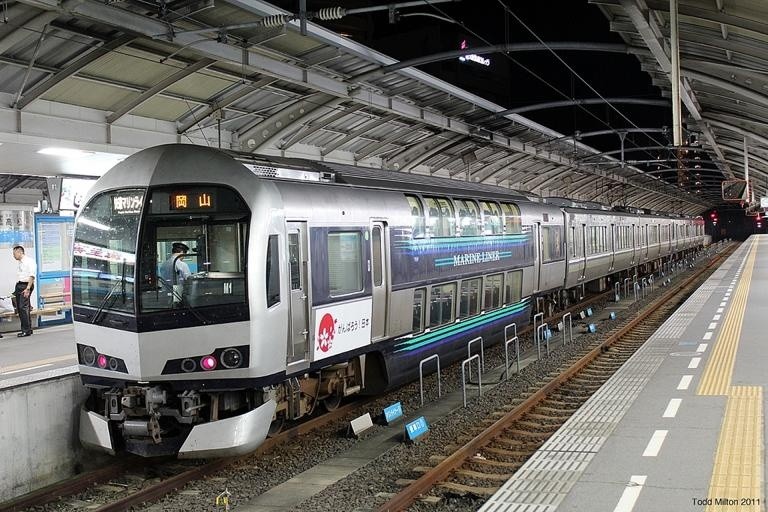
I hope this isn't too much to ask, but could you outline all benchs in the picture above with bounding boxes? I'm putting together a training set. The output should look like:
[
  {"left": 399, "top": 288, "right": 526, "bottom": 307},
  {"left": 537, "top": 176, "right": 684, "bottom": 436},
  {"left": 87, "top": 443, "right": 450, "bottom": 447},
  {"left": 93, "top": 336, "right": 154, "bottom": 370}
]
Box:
[{"left": 0, "top": 291, "right": 71, "bottom": 338}]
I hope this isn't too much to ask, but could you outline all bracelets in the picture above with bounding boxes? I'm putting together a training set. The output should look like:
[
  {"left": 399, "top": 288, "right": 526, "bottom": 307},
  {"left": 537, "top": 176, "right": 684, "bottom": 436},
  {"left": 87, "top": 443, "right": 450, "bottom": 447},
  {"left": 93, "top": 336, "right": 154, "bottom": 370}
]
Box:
[{"left": 27, "top": 288, "right": 30, "bottom": 290}]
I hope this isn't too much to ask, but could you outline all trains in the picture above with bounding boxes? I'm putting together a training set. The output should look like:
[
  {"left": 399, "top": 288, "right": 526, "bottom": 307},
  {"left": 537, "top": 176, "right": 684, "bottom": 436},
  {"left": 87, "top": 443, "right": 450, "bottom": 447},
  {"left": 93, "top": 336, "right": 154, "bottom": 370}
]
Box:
[{"left": 67, "top": 143, "right": 707, "bottom": 463}]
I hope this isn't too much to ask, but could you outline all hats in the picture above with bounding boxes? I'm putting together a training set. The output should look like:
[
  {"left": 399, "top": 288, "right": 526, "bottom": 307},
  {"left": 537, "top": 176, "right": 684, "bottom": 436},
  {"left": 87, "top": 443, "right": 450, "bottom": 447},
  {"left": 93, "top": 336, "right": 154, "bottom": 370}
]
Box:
[{"left": 173, "top": 242, "right": 189, "bottom": 250}]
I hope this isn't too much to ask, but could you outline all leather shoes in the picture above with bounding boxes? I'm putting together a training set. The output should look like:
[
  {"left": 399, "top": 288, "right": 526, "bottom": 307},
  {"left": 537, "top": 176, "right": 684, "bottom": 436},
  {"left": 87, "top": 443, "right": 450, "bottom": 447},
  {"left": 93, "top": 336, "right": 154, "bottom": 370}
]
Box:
[{"left": 18, "top": 332, "right": 33, "bottom": 337}]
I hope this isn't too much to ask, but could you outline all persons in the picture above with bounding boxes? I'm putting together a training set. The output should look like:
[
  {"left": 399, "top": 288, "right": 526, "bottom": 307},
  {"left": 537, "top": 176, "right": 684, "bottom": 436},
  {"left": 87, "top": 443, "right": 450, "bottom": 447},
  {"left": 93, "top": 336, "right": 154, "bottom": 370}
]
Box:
[
  {"left": 159, "top": 242, "right": 192, "bottom": 289},
  {"left": 12, "top": 246, "right": 36, "bottom": 337}
]
